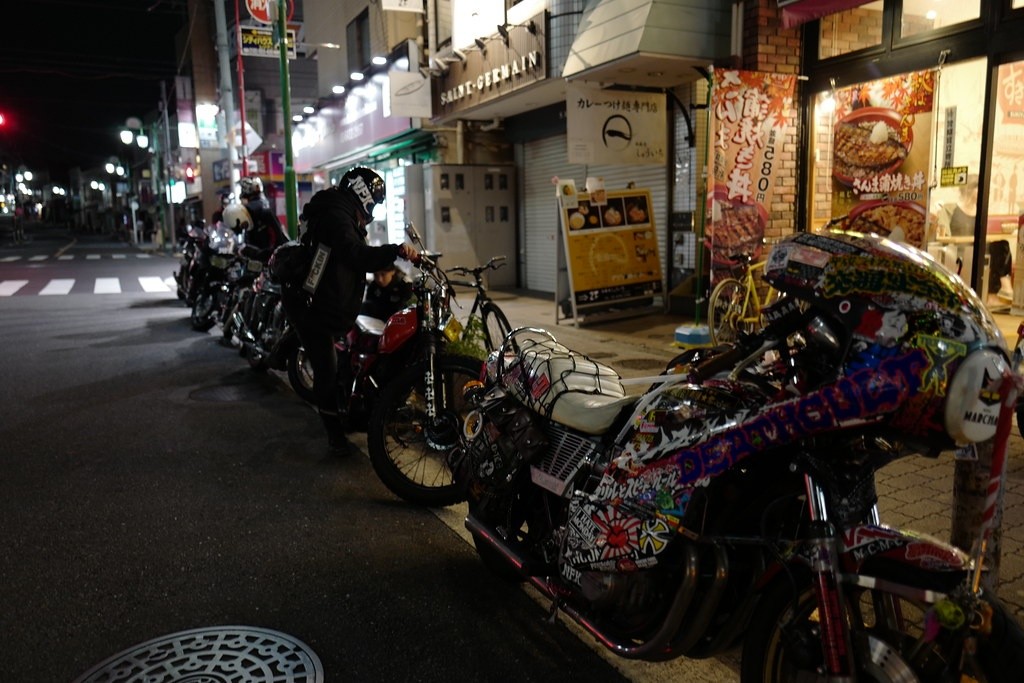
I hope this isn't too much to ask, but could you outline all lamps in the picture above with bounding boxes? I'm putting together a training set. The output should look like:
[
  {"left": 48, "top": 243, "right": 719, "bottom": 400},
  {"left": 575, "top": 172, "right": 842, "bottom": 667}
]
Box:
[
  {"left": 474, "top": 36, "right": 510, "bottom": 50},
  {"left": 452, "top": 47, "right": 474, "bottom": 62},
  {"left": 434, "top": 57, "right": 460, "bottom": 70},
  {"left": 496, "top": 18, "right": 536, "bottom": 38},
  {"left": 419, "top": 66, "right": 443, "bottom": 79}
]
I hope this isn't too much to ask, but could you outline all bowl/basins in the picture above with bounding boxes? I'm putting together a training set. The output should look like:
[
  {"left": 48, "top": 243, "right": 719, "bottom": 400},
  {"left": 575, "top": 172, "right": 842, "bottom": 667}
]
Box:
[
  {"left": 706, "top": 185, "right": 766, "bottom": 265},
  {"left": 832, "top": 107, "right": 913, "bottom": 187},
  {"left": 845, "top": 201, "right": 925, "bottom": 243}
]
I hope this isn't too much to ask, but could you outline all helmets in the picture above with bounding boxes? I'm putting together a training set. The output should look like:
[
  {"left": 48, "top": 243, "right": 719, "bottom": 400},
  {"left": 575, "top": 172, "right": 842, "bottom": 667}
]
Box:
[
  {"left": 239, "top": 175, "right": 263, "bottom": 198},
  {"left": 339, "top": 167, "right": 385, "bottom": 223},
  {"left": 222, "top": 204, "right": 253, "bottom": 232}
]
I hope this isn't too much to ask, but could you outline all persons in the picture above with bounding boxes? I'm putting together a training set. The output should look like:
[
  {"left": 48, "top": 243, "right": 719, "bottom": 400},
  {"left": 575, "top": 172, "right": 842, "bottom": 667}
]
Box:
[
  {"left": 189, "top": 177, "right": 289, "bottom": 330},
  {"left": 281, "top": 167, "right": 417, "bottom": 453}
]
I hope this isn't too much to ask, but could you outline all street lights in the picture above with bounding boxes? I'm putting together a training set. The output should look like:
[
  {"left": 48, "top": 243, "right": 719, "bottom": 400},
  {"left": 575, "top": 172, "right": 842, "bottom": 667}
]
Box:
[
  {"left": 106, "top": 161, "right": 139, "bottom": 245},
  {"left": 91, "top": 179, "right": 112, "bottom": 239},
  {"left": 16, "top": 172, "right": 34, "bottom": 241},
  {"left": 119, "top": 126, "right": 165, "bottom": 250}
]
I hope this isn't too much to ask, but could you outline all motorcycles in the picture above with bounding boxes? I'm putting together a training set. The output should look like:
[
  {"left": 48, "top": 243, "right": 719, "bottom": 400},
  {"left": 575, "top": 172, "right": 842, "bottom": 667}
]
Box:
[
  {"left": 173, "top": 215, "right": 291, "bottom": 373},
  {"left": 268, "top": 223, "right": 500, "bottom": 505},
  {"left": 448, "top": 229, "right": 1024, "bottom": 683}
]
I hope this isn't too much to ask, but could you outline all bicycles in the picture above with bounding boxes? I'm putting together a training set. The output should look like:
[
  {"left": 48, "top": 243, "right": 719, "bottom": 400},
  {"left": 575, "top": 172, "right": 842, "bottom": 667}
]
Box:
[
  {"left": 418, "top": 249, "right": 519, "bottom": 357},
  {"left": 708, "top": 231, "right": 787, "bottom": 349}
]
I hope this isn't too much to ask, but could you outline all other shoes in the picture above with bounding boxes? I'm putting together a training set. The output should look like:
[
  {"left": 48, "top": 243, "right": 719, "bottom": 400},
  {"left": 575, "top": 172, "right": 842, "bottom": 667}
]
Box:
[{"left": 327, "top": 438, "right": 358, "bottom": 458}]
